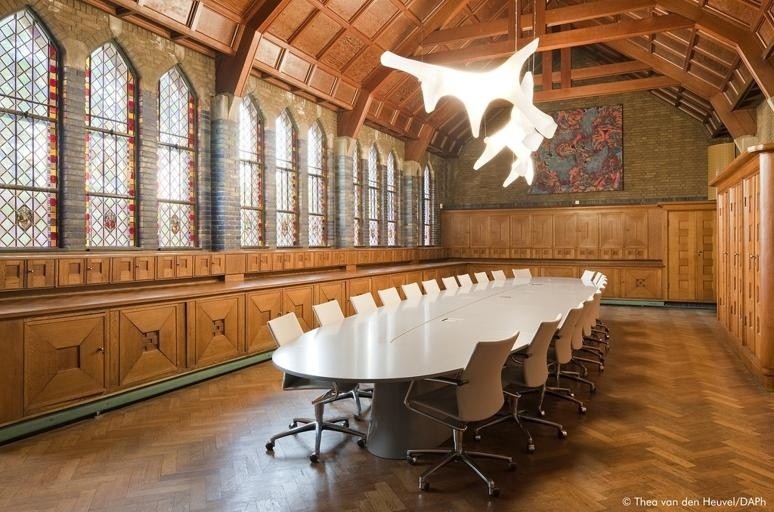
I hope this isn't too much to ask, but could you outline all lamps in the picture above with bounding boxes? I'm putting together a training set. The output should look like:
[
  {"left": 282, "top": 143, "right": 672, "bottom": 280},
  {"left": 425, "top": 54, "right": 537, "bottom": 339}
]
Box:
[{"left": 381, "top": 0, "right": 558, "bottom": 188}]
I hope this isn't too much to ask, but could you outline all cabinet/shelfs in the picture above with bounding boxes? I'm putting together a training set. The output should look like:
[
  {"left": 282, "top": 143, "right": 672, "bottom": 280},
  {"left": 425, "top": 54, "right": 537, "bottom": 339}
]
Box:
[
  {"left": 441, "top": 206, "right": 667, "bottom": 304},
  {"left": 0, "top": 242, "right": 444, "bottom": 444},
  {"left": 708, "top": 143, "right": 773, "bottom": 392}
]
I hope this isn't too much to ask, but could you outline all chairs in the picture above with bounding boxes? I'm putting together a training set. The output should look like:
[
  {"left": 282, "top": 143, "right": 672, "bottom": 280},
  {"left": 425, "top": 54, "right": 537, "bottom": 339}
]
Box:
[{"left": 266, "top": 267, "right": 610, "bottom": 501}]
